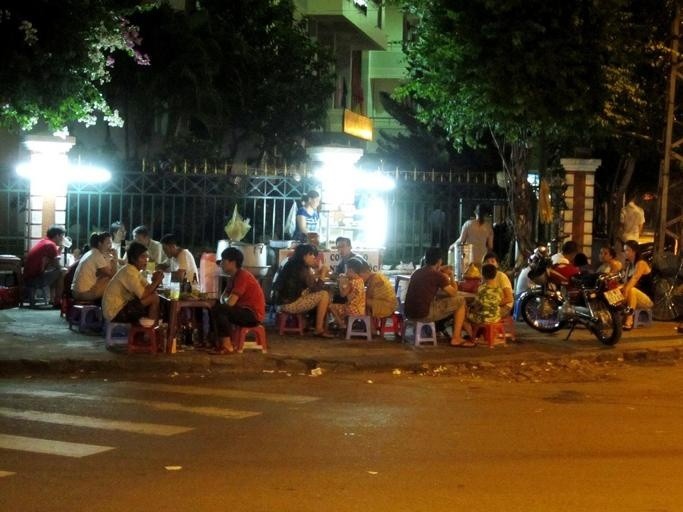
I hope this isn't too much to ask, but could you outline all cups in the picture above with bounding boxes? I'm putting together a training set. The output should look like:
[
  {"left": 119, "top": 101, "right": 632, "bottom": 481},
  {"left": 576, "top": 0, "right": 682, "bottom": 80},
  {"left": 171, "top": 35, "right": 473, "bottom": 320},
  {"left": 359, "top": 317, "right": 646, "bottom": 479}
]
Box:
[
  {"left": 170, "top": 283, "right": 180, "bottom": 300},
  {"left": 162, "top": 272, "right": 171, "bottom": 289},
  {"left": 62, "top": 236, "right": 72, "bottom": 249}
]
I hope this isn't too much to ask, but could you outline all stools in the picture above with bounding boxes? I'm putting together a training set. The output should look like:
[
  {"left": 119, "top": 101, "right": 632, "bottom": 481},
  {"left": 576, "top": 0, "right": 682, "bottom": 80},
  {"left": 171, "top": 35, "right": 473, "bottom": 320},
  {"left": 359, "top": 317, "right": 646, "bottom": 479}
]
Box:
[
  {"left": 375, "top": 311, "right": 402, "bottom": 339},
  {"left": 402, "top": 319, "right": 438, "bottom": 346},
  {"left": 68, "top": 305, "right": 100, "bottom": 334},
  {"left": 231, "top": 325, "right": 269, "bottom": 352},
  {"left": 500, "top": 318, "right": 514, "bottom": 338},
  {"left": 126, "top": 326, "right": 160, "bottom": 353},
  {"left": 277, "top": 312, "right": 304, "bottom": 336},
  {"left": 470, "top": 324, "right": 507, "bottom": 348},
  {"left": 104, "top": 323, "right": 131, "bottom": 349},
  {"left": 344, "top": 315, "right": 372, "bottom": 341},
  {"left": 512, "top": 295, "right": 654, "bottom": 329},
  {"left": 19, "top": 286, "right": 49, "bottom": 308}
]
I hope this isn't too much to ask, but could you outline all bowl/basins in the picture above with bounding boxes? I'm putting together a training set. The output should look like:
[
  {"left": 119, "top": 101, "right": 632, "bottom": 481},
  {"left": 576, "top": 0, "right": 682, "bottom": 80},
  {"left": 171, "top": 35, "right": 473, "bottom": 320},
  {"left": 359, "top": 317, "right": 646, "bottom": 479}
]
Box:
[
  {"left": 139, "top": 318, "right": 155, "bottom": 327},
  {"left": 415, "top": 265, "right": 421, "bottom": 270},
  {"left": 244, "top": 266, "right": 272, "bottom": 277}
]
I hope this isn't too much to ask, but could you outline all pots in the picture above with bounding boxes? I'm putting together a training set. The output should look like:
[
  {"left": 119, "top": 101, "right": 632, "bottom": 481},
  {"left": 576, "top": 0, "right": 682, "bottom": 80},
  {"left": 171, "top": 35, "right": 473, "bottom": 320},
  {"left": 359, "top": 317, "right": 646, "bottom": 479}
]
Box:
[
  {"left": 231, "top": 242, "right": 267, "bottom": 266},
  {"left": 270, "top": 239, "right": 301, "bottom": 249},
  {"left": 215, "top": 239, "right": 229, "bottom": 264}
]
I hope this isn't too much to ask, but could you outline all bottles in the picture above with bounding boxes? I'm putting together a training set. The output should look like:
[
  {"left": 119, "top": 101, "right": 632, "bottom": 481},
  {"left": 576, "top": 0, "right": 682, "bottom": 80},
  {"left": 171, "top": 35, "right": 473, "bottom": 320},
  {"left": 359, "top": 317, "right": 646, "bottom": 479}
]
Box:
[
  {"left": 180, "top": 319, "right": 200, "bottom": 346},
  {"left": 183, "top": 281, "right": 192, "bottom": 295},
  {"left": 337, "top": 206, "right": 345, "bottom": 226},
  {"left": 448, "top": 247, "right": 454, "bottom": 266},
  {"left": 121, "top": 240, "right": 125, "bottom": 258}
]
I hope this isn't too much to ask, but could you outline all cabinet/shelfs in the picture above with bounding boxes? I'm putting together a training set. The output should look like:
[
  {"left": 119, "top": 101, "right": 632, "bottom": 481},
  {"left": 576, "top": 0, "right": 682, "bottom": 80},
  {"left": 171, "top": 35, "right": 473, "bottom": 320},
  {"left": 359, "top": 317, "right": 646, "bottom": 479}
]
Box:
[{"left": 273, "top": 203, "right": 385, "bottom": 273}]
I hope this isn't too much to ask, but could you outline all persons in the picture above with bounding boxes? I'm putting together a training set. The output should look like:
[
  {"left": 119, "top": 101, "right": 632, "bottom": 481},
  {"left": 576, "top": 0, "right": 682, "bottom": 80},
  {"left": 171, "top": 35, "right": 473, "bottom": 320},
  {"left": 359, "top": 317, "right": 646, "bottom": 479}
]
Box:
[
  {"left": 463, "top": 265, "right": 504, "bottom": 343},
  {"left": 327, "top": 237, "right": 363, "bottom": 279},
  {"left": 102, "top": 242, "right": 164, "bottom": 324},
  {"left": 66, "top": 219, "right": 163, "bottom": 304},
  {"left": 357, "top": 257, "right": 397, "bottom": 318},
  {"left": 329, "top": 258, "right": 365, "bottom": 328},
  {"left": 404, "top": 246, "right": 476, "bottom": 349},
  {"left": 160, "top": 234, "right": 199, "bottom": 285},
  {"left": 619, "top": 240, "right": 656, "bottom": 330},
  {"left": 211, "top": 248, "right": 266, "bottom": 356},
  {"left": 518, "top": 241, "right": 622, "bottom": 302},
  {"left": 268, "top": 190, "right": 333, "bottom": 339},
  {"left": 618, "top": 190, "right": 645, "bottom": 241},
  {"left": 24, "top": 226, "right": 67, "bottom": 309},
  {"left": 427, "top": 202, "right": 515, "bottom": 317}
]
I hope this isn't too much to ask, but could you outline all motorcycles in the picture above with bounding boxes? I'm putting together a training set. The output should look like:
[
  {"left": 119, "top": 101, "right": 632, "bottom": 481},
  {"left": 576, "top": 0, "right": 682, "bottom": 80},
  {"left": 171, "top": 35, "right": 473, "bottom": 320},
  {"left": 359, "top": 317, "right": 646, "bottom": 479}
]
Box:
[{"left": 521, "top": 233, "right": 627, "bottom": 345}]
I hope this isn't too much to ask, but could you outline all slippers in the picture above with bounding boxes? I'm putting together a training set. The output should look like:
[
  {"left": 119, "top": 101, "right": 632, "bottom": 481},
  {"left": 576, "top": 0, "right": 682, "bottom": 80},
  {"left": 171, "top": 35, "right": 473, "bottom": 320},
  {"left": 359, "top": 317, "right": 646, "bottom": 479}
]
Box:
[
  {"left": 450, "top": 339, "right": 477, "bottom": 347},
  {"left": 208, "top": 346, "right": 234, "bottom": 355},
  {"left": 313, "top": 331, "right": 335, "bottom": 338},
  {"left": 623, "top": 322, "right": 634, "bottom": 330}
]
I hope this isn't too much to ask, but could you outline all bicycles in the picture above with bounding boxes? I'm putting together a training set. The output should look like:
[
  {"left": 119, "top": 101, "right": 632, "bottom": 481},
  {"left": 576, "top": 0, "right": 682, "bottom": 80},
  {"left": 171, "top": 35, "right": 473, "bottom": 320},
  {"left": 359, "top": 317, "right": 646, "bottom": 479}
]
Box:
[{"left": 645, "top": 249, "right": 683, "bottom": 322}]
